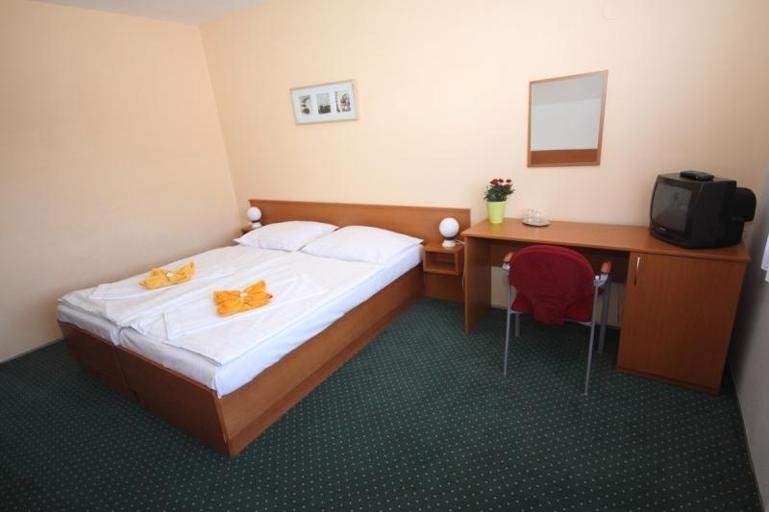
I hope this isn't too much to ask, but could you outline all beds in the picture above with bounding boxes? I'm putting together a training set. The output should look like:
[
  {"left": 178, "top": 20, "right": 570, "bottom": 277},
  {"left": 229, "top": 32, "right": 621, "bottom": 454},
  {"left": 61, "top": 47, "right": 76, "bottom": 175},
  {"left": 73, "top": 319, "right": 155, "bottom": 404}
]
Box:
[{"left": 55, "top": 197, "right": 469, "bottom": 457}]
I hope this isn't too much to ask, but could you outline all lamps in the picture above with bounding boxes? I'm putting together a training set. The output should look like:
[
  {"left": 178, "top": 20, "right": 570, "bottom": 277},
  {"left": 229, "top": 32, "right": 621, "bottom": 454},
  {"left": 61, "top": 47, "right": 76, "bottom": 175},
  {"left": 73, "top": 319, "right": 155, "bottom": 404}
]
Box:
[
  {"left": 439, "top": 217, "right": 460, "bottom": 248},
  {"left": 247, "top": 206, "right": 262, "bottom": 229}
]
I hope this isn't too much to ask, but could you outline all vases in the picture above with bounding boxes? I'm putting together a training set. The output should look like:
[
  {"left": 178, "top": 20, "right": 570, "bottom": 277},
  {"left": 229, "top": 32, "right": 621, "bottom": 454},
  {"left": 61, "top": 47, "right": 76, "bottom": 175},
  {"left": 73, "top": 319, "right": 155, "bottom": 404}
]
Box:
[{"left": 487, "top": 201, "right": 506, "bottom": 224}]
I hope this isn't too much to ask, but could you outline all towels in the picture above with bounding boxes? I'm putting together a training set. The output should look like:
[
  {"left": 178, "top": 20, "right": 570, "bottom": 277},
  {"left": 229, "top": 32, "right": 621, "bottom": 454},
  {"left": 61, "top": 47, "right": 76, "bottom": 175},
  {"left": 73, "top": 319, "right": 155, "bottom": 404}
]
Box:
[
  {"left": 214, "top": 280, "right": 273, "bottom": 312},
  {"left": 138, "top": 261, "right": 193, "bottom": 289}
]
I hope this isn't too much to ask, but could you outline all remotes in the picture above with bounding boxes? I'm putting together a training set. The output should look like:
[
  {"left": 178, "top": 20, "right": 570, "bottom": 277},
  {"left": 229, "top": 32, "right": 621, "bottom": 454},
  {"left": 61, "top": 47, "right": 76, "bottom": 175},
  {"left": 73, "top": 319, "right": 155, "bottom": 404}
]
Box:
[{"left": 681, "top": 170, "right": 714, "bottom": 181}]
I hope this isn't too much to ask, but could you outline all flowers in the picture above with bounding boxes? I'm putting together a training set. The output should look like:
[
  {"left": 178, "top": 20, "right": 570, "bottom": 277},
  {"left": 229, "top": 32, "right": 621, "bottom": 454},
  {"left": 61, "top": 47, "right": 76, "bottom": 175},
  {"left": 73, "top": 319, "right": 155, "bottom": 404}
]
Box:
[{"left": 482, "top": 178, "right": 515, "bottom": 201}]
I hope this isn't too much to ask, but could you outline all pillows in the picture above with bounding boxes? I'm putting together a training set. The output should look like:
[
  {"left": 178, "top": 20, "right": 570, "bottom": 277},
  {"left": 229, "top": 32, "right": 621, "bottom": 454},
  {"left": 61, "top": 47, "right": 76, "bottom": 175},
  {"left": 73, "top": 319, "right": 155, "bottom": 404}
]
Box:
[
  {"left": 300, "top": 224, "right": 424, "bottom": 265},
  {"left": 232, "top": 220, "right": 340, "bottom": 252}
]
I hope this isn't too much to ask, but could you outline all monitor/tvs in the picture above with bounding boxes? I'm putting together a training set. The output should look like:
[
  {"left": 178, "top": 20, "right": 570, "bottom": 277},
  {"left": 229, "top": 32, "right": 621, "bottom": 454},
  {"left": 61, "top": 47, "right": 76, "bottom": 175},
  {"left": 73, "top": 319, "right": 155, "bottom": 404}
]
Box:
[{"left": 647, "top": 173, "right": 757, "bottom": 249}]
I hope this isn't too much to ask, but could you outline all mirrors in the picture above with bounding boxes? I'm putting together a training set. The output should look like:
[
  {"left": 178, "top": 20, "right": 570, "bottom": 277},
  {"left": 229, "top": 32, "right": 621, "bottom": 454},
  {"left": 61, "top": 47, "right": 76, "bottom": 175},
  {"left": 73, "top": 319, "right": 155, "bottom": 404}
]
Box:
[{"left": 527, "top": 69, "right": 607, "bottom": 167}]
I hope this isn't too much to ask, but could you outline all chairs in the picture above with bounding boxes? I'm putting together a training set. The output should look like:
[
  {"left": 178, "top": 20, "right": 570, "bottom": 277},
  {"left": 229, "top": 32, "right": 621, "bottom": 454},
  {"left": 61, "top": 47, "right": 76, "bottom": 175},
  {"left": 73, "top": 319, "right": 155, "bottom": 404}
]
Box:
[{"left": 501, "top": 245, "right": 612, "bottom": 398}]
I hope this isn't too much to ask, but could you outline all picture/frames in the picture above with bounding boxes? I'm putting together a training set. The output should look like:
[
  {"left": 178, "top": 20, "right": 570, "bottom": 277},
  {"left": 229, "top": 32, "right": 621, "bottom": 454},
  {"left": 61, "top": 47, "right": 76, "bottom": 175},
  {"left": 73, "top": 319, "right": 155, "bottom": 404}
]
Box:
[{"left": 289, "top": 80, "right": 359, "bottom": 125}]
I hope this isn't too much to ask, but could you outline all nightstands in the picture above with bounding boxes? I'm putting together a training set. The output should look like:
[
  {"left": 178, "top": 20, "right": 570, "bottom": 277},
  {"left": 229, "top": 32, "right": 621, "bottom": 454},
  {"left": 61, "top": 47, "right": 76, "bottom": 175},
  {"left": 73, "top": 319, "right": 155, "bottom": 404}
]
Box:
[
  {"left": 241, "top": 226, "right": 252, "bottom": 234},
  {"left": 423, "top": 241, "right": 464, "bottom": 276}
]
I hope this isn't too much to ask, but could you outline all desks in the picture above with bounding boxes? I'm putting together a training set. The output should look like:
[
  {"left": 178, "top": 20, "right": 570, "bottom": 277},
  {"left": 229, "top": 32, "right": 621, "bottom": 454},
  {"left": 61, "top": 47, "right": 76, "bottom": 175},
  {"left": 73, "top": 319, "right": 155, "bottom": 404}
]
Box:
[{"left": 460, "top": 217, "right": 752, "bottom": 396}]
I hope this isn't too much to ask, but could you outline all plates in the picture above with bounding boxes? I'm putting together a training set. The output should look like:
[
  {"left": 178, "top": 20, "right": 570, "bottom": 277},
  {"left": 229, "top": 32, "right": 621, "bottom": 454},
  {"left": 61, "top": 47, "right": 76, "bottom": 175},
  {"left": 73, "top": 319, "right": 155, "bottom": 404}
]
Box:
[{"left": 522, "top": 219, "right": 551, "bottom": 227}]
